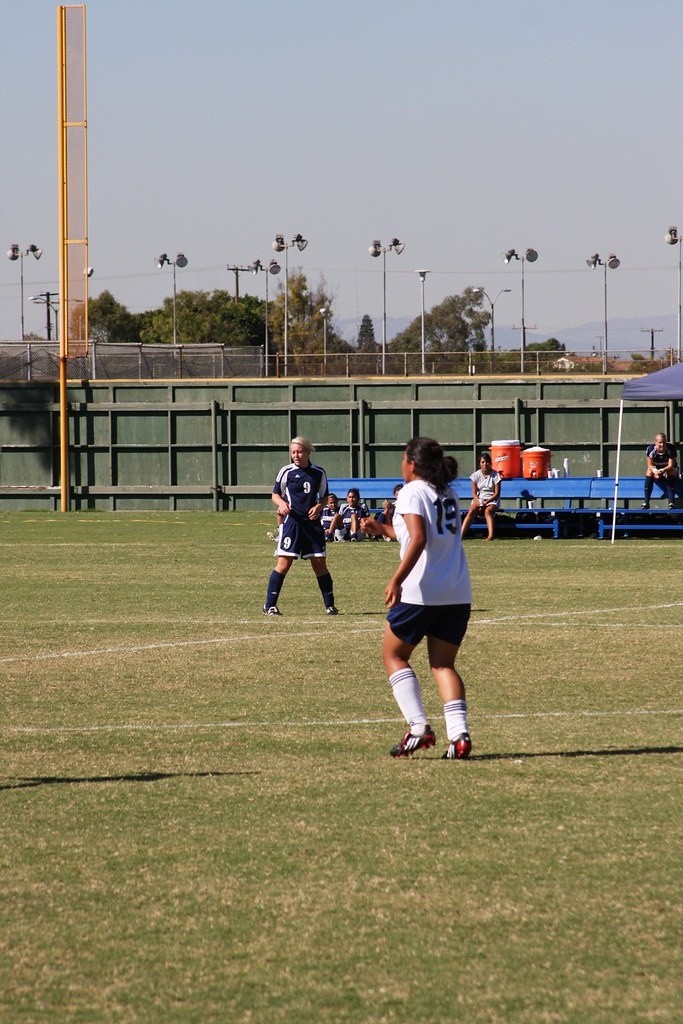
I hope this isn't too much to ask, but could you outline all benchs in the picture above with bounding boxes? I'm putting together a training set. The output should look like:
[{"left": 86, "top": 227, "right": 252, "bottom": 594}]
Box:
[{"left": 322, "top": 478, "right": 683, "bottom": 540}]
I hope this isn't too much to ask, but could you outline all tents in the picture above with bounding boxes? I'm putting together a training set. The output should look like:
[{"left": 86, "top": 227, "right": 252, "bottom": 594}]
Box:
[{"left": 612, "top": 362, "right": 683, "bottom": 544}]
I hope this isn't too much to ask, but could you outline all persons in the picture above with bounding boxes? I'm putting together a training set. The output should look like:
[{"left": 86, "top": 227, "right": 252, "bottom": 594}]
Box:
[
  {"left": 641, "top": 433, "right": 679, "bottom": 508},
  {"left": 462, "top": 453, "right": 502, "bottom": 541},
  {"left": 262, "top": 436, "right": 339, "bottom": 616},
  {"left": 267, "top": 484, "right": 404, "bottom": 543},
  {"left": 381, "top": 437, "right": 473, "bottom": 759}
]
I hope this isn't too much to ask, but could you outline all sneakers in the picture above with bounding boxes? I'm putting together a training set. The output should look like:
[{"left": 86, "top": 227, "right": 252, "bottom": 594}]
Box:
[
  {"left": 263, "top": 603, "right": 282, "bottom": 616},
  {"left": 327, "top": 607, "right": 345, "bottom": 616},
  {"left": 441, "top": 733, "right": 472, "bottom": 759},
  {"left": 640, "top": 502, "right": 650, "bottom": 509},
  {"left": 668, "top": 503, "right": 676, "bottom": 509},
  {"left": 390, "top": 725, "right": 436, "bottom": 759}
]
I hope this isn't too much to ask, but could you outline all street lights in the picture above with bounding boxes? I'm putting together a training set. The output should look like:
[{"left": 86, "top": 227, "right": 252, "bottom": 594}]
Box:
[
  {"left": 154, "top": 252, "right": 189, "bottom": 345},
  {"left": 504, "top": 247, "right": 538, "bottom": 373},
  {"left": 585, "top": 251, "right": 621, "bottom": 375},
  {"left": 29, "top": 296, "right": 84, "bottom": 340},
  {"left": 368, "top": 237, "right": 406, "bottom": 376},
  {"left": 664, "top": 226, "right": 683, "bottom": 363},
  {"left": 247, "top": 259, "right": 281, "bottom": 377},
  {"left": 414, "top": 268, "right": 433, "bottom": 375},
  {"left": 7, "top": 244, "right": 43, "bottom": 340},
  {"left": 472, "top": 286, "right": 512, "bottom": 375},
  {"left": 272, "top": 233, "right": 308, "bottom": 376}
]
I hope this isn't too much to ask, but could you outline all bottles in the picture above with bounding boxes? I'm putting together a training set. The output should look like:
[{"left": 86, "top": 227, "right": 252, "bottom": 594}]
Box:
[{"left": 653, "top": 466, "right": 659, "bottom": 478}]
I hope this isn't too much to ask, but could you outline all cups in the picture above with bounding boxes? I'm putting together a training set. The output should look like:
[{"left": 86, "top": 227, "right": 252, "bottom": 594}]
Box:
[
  {"left": 528, "top": 501, "right": 534, "bottom": 509},
  {"left": 564, "top": 458, "right": 569, "bottom": 478},
  {"left": 552, "top": 468, "right": 556, "bottom": 477},
  {"left": 597, "top": 470, "right": 603, "bottom": 477},
  {"left": 479, "top": 499, "right": 484, "bottom": 506},
  {"left": 548, "top": 471, "right": 553, "bottom": 478},
  {"left": 534, "top": 536, "right": 542, "bottom": 542},
  {"left": 555, "top": 470, "right": 560, "bottom": 478}
]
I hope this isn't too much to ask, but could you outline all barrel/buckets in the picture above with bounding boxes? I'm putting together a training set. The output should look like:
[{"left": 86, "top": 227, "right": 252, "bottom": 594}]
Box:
[
  {"left": 489, "top": 446, "right": 522, "bottom": 478},
  {"left": 521, "top": 451, "right": 553, "bottom": 478}
]
